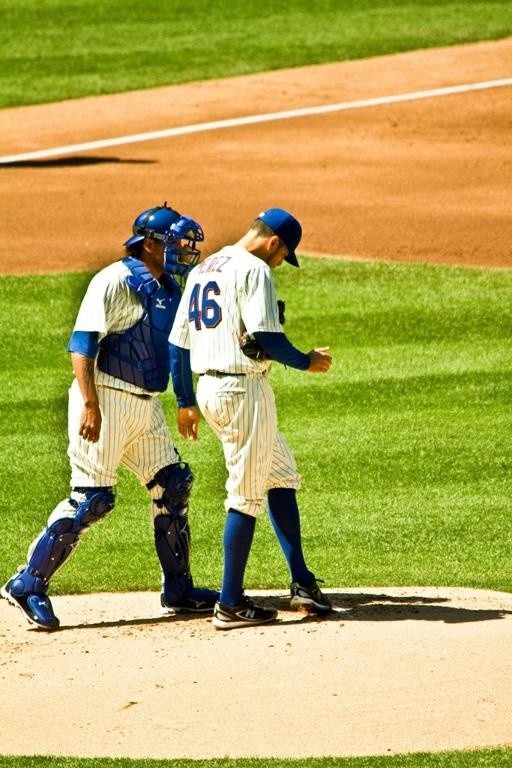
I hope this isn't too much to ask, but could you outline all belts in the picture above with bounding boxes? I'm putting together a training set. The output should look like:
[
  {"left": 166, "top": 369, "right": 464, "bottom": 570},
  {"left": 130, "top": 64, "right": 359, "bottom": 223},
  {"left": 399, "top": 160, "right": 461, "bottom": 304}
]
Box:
[
  {"left": 104, "top": 385, "right": 152, "bottom": 400},
  {"left": 198, "top": 369, "right": 266, "bottom": 377}
]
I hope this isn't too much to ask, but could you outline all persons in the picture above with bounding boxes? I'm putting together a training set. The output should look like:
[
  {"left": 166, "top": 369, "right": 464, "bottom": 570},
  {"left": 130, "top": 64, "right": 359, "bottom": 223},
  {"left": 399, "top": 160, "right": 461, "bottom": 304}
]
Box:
[
  {"left": 167, "top": 208, "right": 336, "bottom": 631},
  {"left": 0, "top": 199, "right": 221, "bottom": 631}
]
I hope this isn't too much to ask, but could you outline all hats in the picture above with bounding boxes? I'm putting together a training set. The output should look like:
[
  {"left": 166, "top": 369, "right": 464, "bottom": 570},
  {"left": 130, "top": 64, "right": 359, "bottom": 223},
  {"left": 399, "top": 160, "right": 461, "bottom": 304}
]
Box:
[{"left": 254, "top": 207, "right": 303, "bottom": 267}]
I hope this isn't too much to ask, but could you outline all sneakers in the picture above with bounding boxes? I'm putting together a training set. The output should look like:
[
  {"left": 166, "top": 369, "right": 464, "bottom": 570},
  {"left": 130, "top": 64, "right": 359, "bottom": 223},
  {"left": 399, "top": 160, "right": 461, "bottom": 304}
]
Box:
[
  {"left": 1, "top": 564, "right": 60, "bottom": 630},
  {"left": 289, "top": 572, "right": 332, "bottom": 612},
  {"left": 160, "top": 589, "right": 223, "bottom": 612},
  {"left": 212, "top": 596, "right": 278, "bottom": 630}
]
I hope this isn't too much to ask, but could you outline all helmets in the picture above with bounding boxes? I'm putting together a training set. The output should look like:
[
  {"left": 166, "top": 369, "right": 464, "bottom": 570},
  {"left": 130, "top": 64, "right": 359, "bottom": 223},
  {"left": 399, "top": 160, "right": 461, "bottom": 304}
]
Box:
[{"left": 128, "top": 203, "right": 205, "bottom": 278}]
